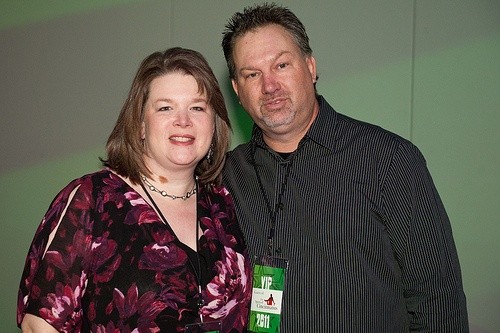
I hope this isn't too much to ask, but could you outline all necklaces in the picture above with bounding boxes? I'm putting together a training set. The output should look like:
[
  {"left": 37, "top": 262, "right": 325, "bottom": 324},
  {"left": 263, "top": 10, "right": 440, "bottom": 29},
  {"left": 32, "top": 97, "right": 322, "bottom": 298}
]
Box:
[{"left": 139, "top": 172, "right": 196, "bottom": 201}]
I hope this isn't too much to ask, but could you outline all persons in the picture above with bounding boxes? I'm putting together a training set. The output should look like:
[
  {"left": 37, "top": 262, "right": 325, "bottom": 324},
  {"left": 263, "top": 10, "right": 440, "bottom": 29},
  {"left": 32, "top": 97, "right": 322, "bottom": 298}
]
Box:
[
  {"left": 16, "top": 46, "right": 253, "bottom": 333},
  {"left": 218, "top": 0, "right": 469, "bottom": 333}
]
